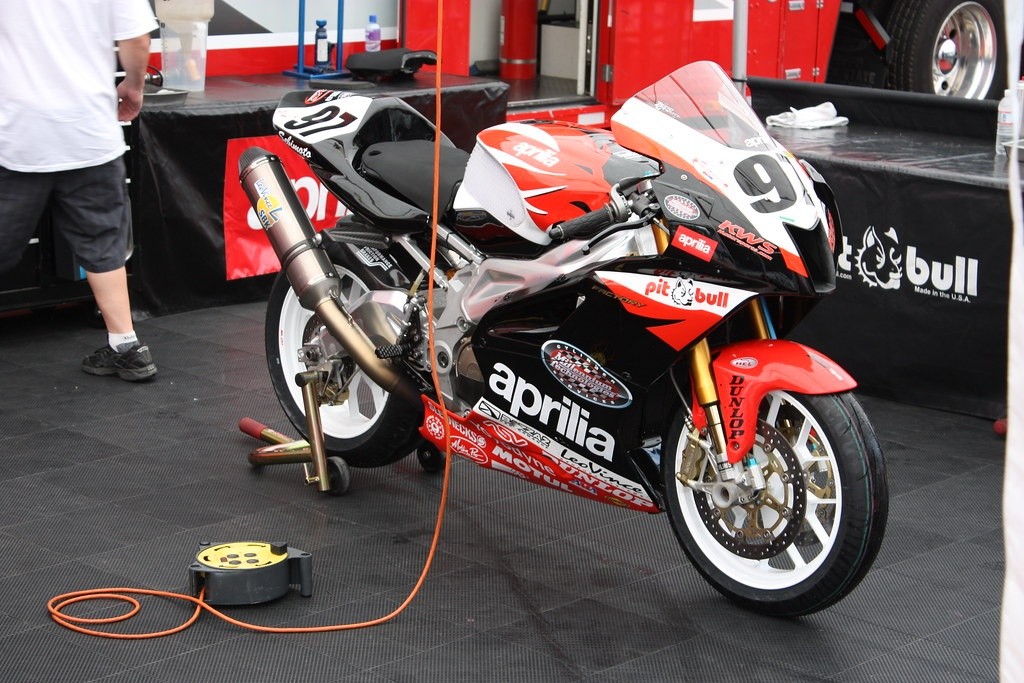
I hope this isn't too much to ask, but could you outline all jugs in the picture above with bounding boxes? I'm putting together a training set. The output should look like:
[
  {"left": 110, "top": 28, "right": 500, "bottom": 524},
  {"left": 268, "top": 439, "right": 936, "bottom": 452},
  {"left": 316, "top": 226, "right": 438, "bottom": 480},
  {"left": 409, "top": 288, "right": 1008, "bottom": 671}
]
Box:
[{"left": 156, "top": 19, "right": 212, "bottom": 92}]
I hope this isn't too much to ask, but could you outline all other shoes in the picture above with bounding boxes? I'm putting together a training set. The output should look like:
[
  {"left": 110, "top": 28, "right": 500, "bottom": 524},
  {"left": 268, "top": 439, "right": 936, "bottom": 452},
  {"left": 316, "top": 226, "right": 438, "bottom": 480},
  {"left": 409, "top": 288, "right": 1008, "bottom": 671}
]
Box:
[{"left": 993, "top": 419, "right": 1006, "bottom": 436}]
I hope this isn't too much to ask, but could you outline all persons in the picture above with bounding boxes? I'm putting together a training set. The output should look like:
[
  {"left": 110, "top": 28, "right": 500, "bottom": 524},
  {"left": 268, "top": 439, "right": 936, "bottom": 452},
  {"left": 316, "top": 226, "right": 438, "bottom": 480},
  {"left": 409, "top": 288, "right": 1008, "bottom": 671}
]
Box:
[{"left": 0, "top": 0, "right": 159, "bottom": 380}]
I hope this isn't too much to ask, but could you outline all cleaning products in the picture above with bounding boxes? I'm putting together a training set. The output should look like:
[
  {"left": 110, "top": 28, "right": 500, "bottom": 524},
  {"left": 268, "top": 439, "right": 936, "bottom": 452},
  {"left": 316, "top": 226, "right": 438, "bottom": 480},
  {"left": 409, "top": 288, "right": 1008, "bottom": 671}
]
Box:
[{"left": 365, "top": 15, "right": 381, "bottom": 52}]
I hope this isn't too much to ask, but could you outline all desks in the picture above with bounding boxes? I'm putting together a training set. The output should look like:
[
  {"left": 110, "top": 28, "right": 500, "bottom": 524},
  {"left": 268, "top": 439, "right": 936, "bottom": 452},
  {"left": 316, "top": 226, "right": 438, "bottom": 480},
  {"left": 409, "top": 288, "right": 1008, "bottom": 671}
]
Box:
[
  {"left": 128, "top": 68, "right": 510, "bottom": 316},
  {"left": 763, "top": 120, "right": 1024, "bottom": 425}
]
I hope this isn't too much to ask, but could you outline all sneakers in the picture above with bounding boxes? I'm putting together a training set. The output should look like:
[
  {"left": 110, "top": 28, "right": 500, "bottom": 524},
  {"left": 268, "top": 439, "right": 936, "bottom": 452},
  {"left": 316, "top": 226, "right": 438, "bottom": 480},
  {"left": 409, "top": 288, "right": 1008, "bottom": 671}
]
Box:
[{"left": 81, "top": 343, "right": 158, "bottom": 381}]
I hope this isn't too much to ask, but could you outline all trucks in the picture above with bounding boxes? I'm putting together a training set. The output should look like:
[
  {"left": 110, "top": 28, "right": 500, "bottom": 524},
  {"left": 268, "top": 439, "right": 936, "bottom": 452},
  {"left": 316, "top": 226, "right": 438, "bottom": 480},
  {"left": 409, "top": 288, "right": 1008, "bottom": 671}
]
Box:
[{"left": 1, "top": 0, "right": 1024, "bottom": 320}]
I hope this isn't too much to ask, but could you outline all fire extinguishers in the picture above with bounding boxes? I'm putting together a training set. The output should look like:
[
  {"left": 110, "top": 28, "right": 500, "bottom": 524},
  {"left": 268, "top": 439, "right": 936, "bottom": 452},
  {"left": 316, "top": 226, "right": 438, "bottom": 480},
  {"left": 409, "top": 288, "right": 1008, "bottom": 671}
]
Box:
[{"left": 497, "top": 0, "right": 539, "bottom": 82}]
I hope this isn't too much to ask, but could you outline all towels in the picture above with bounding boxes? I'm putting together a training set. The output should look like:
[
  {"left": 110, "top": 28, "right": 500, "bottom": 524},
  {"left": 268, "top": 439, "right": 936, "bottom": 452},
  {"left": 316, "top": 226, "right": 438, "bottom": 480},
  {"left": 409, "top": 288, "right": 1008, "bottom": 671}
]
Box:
[{"left": 766, "top": 101, "right": 849, "bottom": 129}]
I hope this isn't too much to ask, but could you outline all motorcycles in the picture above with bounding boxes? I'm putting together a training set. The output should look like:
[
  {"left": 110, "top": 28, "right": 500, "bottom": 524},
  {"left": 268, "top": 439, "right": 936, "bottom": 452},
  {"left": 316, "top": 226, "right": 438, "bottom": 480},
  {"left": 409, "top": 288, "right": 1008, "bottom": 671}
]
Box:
[{"left": 234, "top": 59, "right": 892, "bottom": 615}]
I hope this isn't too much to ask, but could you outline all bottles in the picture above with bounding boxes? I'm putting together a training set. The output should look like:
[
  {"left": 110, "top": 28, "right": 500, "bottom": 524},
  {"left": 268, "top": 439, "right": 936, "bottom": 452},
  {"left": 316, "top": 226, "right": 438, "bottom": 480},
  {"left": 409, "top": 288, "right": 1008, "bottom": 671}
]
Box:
[
  {"left": 995, "top": 89, "right": 1019, "bottom": 155},
  {"left": 365, "top": 15, "right": 382, "bottom": 52}
]
